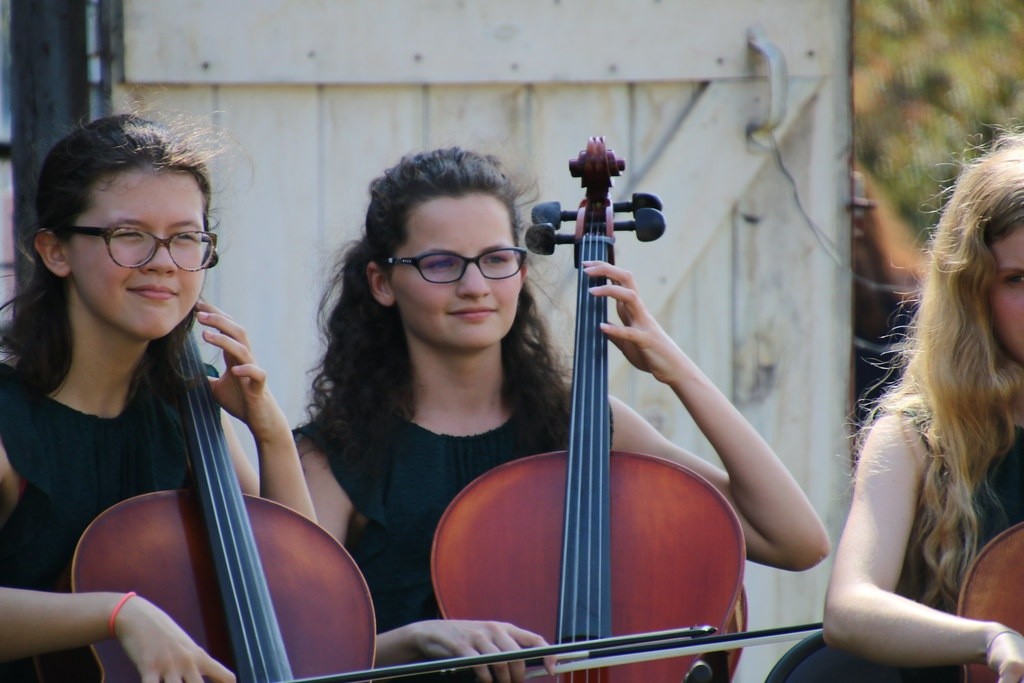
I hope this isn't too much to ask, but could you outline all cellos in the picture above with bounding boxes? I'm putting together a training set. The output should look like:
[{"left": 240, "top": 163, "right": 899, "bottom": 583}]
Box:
[
  {"left": 433, "top": 134, "right": 751, "bottom": 683},
  {"left": 37, "top": 319, "right": 376, "bottom": 683},
  {"left": 954, "top": 520, "right": 1024, "bottom": 683}
]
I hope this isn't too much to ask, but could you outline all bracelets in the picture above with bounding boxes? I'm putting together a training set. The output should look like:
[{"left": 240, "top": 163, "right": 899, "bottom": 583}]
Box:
[
  {"left": 109, "top": 591, "right": 136, "bottom": 640},
  {"left": 984, "top": 630, "right": 1022, "bottom": 654}
]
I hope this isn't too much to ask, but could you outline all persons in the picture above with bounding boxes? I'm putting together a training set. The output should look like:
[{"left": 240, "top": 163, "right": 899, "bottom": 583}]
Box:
[
  {"left": 821, "top": 130, "right": 1024, "bottom": 682},
  {"left": 291, "top": 149, "right": 833, "bottom": 683},
  {"left": 1, "top": 113, "right": 322, "bottom": 683}
]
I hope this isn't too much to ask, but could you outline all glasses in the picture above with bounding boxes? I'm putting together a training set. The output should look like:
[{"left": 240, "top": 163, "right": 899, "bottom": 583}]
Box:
[
  {"left": 52, "top": 226, "right": 218, "bottom": 272},
  {"left": 382, "top": 248, "right": 527, "bottom": 283}
]
